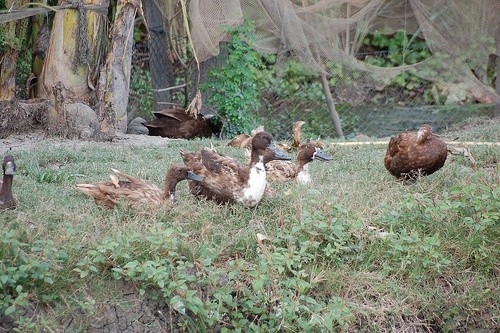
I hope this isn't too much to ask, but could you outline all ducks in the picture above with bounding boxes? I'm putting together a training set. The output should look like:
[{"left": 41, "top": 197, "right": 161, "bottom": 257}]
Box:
[
  {"left": 0, "top": 154, "right": 19, "bottom": 211},
  {"left": 73, "top": 163, "right": 205, "bottom": 220},
  {"left": 179, "top": 116, "right": 334, "bottom": 211},
  {"left": 383, "top": 124, "right": 448, "bottom": 183}
]
